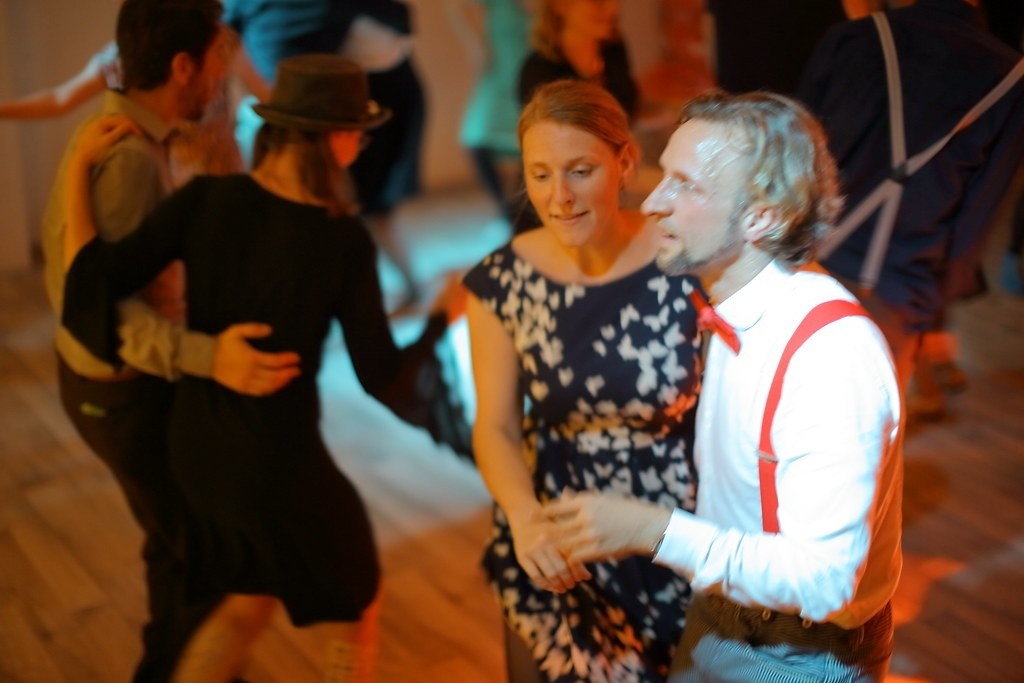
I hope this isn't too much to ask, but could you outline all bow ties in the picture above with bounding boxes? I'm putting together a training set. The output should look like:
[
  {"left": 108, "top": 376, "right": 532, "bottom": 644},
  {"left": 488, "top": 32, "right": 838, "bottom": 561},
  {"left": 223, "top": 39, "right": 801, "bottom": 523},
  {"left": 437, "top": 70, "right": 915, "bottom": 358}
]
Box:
[{"left": 690, "top": 289, "right": 741, "bottom": 355}]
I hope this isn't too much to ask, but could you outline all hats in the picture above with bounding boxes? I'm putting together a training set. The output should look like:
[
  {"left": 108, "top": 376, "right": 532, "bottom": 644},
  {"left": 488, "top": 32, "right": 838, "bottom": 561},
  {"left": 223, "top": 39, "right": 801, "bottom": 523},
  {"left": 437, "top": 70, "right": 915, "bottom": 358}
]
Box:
[{"left": 251, "top": 53, "right": 393, "bottom": 131}]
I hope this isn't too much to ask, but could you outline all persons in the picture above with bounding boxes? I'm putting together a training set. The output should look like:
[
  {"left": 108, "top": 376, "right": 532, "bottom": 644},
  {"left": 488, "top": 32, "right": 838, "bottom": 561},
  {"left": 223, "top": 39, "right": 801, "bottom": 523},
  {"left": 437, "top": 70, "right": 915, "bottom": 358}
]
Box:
[
  {"left": 505, "top": 1, "right": 643, "bottom": 240},
  {"left": 217, "top": 0, "right": 333, "bottom": 166},
  {"left": 333, "top": 1, "right": 428, "bottom": 320},
  {"left": 1, "top": 25, "right": 274, "bottom": 178},
  {"left": 699, "top": 0, "right": 1024, "bottom": 419},
  {"left": 539, "top": 90, "right": 905, "bottom": 683},
  {"left": 45, "top": 1, "right": 302, "bottom": 683},
  {"left": 60, "top": 56, "right": 469, "bottom": 683},
  {"left": 460, "top": 79, "right": 712, "bottom": 683}
]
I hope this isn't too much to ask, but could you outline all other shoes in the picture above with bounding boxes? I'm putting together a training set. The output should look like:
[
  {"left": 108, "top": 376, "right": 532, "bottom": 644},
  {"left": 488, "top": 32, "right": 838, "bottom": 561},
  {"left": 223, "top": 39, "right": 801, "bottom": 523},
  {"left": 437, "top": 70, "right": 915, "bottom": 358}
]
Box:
[
  {"left": 394, "top": 289, "right": 424, "bottom": 310},
  {"left": 408, "top": 344, "right": 443, "bottom": 369},
  {"left": 930, "top": 348, "right": 970, "bottom": 393},
  {"left": 904, "top": 377, "right": 949, "bottom": 424}
]
